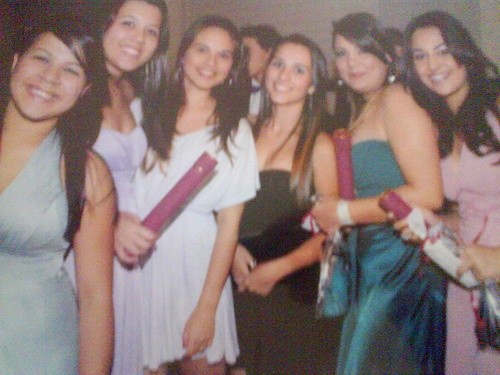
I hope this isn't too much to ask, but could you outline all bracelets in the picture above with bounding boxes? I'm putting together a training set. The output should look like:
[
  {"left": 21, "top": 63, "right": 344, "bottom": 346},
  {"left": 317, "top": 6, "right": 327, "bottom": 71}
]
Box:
[{"left": 338, "top": 198, "right": 354, "bottom": 228}]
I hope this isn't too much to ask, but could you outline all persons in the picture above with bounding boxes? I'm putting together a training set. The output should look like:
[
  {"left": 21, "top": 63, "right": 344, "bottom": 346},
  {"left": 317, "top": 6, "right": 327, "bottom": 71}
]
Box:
[
  {"left": 0, "top": 0, "right": 499, "bottom": 374},
  {"left": 236, "top": 21, "right": 283, "bottom": 127}
]
